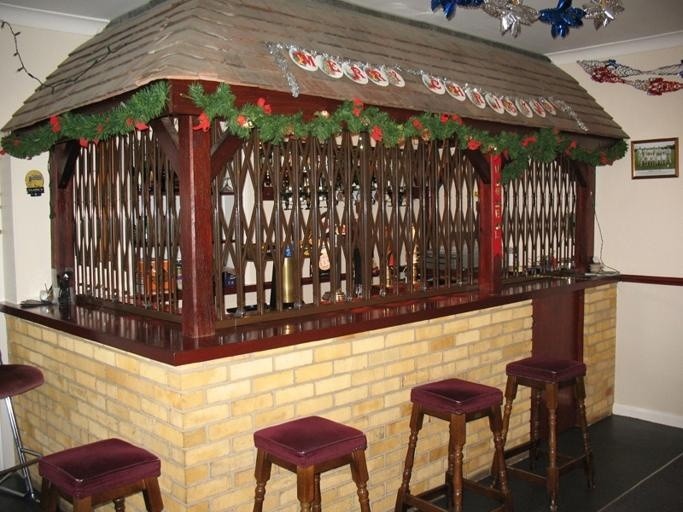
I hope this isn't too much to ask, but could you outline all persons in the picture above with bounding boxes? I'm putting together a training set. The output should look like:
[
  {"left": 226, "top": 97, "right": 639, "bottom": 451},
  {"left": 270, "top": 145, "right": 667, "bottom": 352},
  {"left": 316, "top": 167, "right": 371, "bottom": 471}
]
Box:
[{"left": 636, "top": 146, "right": 672, "bottom": 169}]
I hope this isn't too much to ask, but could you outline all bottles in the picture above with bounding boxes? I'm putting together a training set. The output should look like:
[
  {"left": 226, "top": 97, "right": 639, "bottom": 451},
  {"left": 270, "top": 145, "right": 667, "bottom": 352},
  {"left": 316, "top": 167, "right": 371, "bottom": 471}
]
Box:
[{"left": 282, "top": 244, "right": 296, "bottom": 305}]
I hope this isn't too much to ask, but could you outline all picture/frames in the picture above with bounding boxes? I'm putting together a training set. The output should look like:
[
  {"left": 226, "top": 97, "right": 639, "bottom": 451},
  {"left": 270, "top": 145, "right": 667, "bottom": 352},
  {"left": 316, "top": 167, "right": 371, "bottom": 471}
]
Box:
[{"left": 630, "top": 137, "right": 681, "bottom": 180}]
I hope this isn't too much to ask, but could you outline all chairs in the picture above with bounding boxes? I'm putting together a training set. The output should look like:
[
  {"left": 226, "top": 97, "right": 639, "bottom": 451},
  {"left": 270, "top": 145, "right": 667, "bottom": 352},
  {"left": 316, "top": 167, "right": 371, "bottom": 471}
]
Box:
[{"left": 0, "top": 349, "right": 45, "bottom": 500}]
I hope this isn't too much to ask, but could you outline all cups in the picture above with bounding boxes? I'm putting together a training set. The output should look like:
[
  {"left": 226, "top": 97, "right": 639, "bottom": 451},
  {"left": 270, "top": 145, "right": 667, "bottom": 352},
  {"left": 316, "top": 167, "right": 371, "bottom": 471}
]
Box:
[{"left": 591, "top": 263, "right": 601, "bottom": 274}]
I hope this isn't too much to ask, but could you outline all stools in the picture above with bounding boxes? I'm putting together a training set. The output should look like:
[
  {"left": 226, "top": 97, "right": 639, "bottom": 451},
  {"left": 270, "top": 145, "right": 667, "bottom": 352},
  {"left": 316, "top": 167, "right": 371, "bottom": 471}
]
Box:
[
  {"left": 252, "top": 415, "right": 371, "bottom": 512},
  {"left": 395, "top": 378, "right": 513, "bottom": 512},
  {"left": 40, "top": 438, "right": 163, "bottom": 512},
  {"left": 492, "top": 356, "right": 595, "bottom": 512}
]
